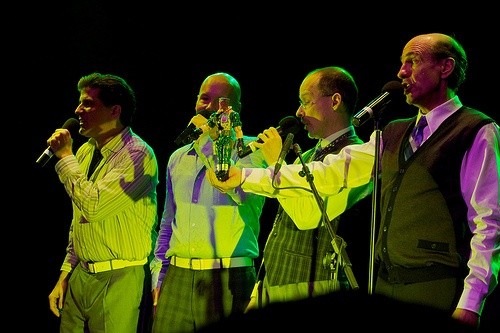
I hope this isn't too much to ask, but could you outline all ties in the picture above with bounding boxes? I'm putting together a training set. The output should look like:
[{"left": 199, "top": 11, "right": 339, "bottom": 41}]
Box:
[{"left": 402, "top": 116, "right": 428, "bottom": 161}]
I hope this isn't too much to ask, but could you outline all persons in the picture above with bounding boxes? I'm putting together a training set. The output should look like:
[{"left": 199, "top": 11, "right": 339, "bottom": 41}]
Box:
[
  {"left": 152, "top": 72, "right": 274, "bottom": 333},
  {"left": 47, "top": 74, "right": 160, "bottom": 333},
  {"left": 243, "top": 65, "right": 374, "bottom": 311},
  {"left": 206, "top": 33, "right": 500, "bottom": 329}
]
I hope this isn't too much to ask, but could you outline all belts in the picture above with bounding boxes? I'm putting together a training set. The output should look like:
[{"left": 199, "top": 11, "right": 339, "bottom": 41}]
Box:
[
  {"left": 169, "top": 254, "right": 254, "bottom": 270},
  {"left": 79, "top": 257, "right": 148, "bottom": 274}
]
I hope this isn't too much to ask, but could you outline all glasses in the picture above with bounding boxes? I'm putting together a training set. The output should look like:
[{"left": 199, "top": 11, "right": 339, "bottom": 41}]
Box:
[{"left": 299, "top": 93, "right": 332, "bottom": 109}]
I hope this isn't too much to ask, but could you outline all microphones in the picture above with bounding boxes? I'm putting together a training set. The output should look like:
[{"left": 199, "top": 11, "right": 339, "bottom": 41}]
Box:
[
  {"left": 35, "top": 118, "right": 81, "bottom": 168},
  {"left": 351, "top": 80, "right": 405, "bottom": 129},
  {"left": 273, "top": 120, "right": 301, "bottom": 175},
  {"left": 236, "top": 115, "right": 297, "bottom": 159},
  {"left": 174, "top": 110, "right": 211, "bottom": 145}
]
